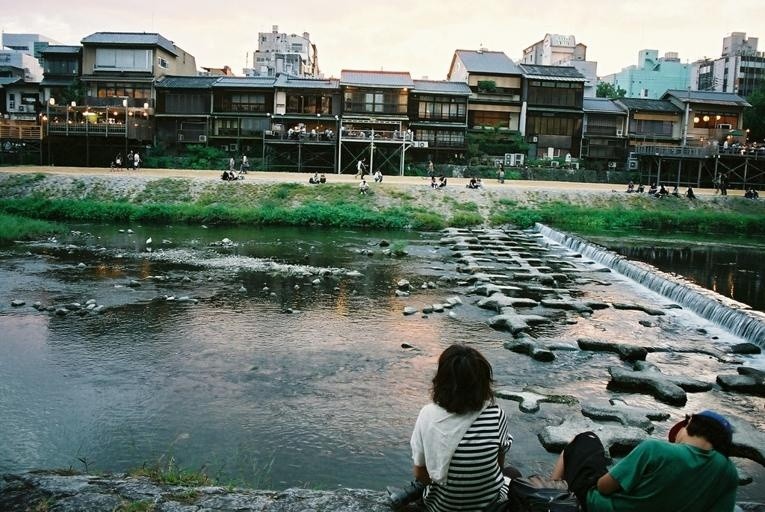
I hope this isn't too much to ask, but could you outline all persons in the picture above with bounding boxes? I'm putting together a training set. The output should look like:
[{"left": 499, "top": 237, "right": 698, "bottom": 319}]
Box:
[
  {"left": 465, "top": 175, "right": 486, "bottom": 189},
  {"left": 220, "top": 152, "right": 249, "bottom": 180},
  {"left": 628, "top": 181, "right": 760, "bottom": 199},
  {"left": 705, "top": 139, "right": 757, "bottom": 156},
  {"left": 110, "top": 150, "right": 142, "bottom": 172},
  {"left": 428, "top": 159, "right": 447, "bottom": 188},
  {"left": 551, "top": 410, "right": 737, "bottom": 512},
  {"left": 389, "top": 345, "right": 523, "bottom": 512},
  {"left": 355, "top": 159, "right": 383, "bottom": 196},
  {"left": 309, "top": 172, "right": 325, "bottom": 184},
  {"left": 495, "top": 163, "right": 504, "bottom": 184},
  {"left": 288, "top": 126, "right": 413, "bottom": 140}
]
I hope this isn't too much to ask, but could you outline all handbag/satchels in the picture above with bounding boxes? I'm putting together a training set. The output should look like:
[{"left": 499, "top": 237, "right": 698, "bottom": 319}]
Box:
[{"left": 507, "top": 477, "right": 579, "bottom": 512}]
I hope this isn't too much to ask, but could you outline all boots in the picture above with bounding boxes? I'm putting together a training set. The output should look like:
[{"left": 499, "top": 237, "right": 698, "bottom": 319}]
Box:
[{"left": 386, "top": 484, "right": 420, "bottom": 510}]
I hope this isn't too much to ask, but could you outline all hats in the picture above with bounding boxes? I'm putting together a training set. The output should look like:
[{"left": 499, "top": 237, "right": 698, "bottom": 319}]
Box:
[{"left": 669, "top": 411, "right": 732, "bottom": 442}]
